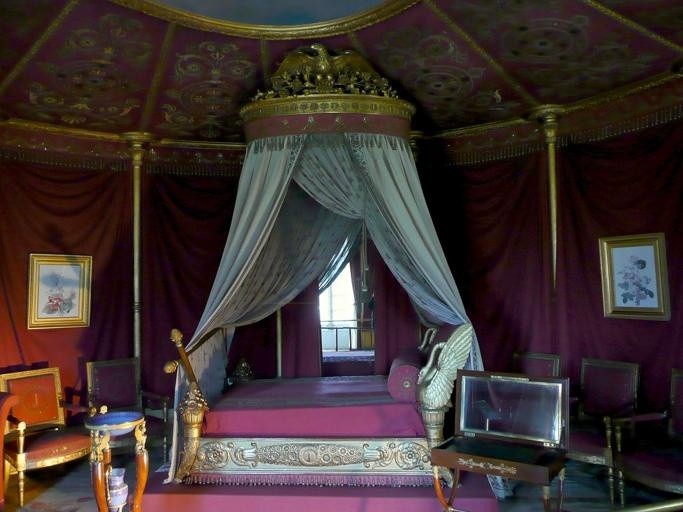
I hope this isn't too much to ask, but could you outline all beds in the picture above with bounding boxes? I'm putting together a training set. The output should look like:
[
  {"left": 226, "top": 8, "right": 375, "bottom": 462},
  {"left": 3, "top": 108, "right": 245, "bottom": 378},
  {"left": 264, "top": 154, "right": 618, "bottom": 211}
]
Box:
[{"left": 164, "top": 325, "right": 473, "bottom": 488}]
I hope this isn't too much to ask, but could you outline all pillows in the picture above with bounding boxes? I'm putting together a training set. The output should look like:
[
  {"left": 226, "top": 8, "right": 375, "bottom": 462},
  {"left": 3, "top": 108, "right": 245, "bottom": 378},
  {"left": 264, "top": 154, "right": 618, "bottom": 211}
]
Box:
[{"left": 387, "top": 346, "right": 424, "bottom": 402}]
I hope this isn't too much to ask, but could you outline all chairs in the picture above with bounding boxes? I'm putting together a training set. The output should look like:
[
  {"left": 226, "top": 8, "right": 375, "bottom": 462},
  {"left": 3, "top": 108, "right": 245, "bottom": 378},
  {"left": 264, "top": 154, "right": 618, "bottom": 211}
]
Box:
[
  {"left": 0, "top": 367, "right": 97, "bottom": 507},
  {"left": 0, "top": 392, "right": 18, "bottom": 507},
  {"left": 85, "top": 356, "right": 170, "bottom": 465},
  {"left": 509, "top": 352, "right": 683, "bottom": 512}
]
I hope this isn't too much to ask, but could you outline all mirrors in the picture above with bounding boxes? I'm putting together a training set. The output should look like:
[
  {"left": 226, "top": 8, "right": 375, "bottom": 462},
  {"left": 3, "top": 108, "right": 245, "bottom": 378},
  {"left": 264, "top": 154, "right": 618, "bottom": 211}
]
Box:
[{"left": 460, "top": 375, "right": 563, "bottom": 445}]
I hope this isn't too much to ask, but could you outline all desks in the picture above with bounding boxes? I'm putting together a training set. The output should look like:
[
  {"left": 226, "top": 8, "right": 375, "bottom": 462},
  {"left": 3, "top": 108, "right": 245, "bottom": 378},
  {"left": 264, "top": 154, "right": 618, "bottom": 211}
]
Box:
[{"left": 430, "top": 369, "right": 569, "bottom": 512}]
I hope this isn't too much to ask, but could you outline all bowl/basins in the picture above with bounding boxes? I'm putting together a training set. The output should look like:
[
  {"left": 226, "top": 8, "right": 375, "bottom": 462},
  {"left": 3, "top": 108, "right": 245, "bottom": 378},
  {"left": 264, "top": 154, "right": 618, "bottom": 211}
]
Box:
[{"left": 84, "top": 410, "right": 143, "bottom": 436}]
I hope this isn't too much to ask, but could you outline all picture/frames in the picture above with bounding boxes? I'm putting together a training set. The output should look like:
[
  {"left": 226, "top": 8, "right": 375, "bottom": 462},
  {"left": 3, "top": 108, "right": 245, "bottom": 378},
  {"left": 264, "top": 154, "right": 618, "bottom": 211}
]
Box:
[
  {"left": 27, "top": 252, "right": 93, "bottom": 330},
  {"left": 598, "top": 231, "right": 671, "bottom": 322}
]
